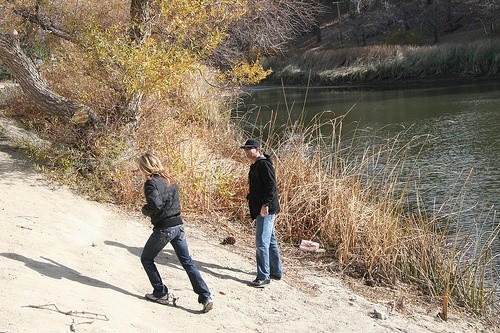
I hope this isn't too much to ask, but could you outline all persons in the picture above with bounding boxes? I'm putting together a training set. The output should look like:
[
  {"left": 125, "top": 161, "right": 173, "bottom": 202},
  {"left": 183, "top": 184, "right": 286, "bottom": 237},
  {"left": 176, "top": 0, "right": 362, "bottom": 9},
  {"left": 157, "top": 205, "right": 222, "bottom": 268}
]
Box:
[
  {"left": 137, "top": 153, "right": 214, "bottom": 313},
  {"left": 240, "top": 139, "right": 283, "bottom": 287}
]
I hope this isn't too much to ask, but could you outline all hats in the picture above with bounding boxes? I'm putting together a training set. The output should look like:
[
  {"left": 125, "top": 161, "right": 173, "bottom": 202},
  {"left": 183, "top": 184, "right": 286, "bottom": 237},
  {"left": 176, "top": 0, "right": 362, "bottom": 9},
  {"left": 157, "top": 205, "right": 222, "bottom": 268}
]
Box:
[{"left": 239, "top": 140, "right": 260, "bottom": 149}]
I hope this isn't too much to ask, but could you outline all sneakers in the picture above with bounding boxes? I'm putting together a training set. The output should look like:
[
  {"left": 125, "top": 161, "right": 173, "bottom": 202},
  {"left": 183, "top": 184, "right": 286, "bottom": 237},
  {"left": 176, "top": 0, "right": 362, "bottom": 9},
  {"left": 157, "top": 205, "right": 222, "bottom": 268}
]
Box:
[
  {"left": 252, "top": 279, "right": 270, "bottom": 288},
  {"left": 146, "top": 294, "right": 169, "bottom": 305},
  {"left": 203, "top": 299, "right": 213, "bottom": 313}
]
